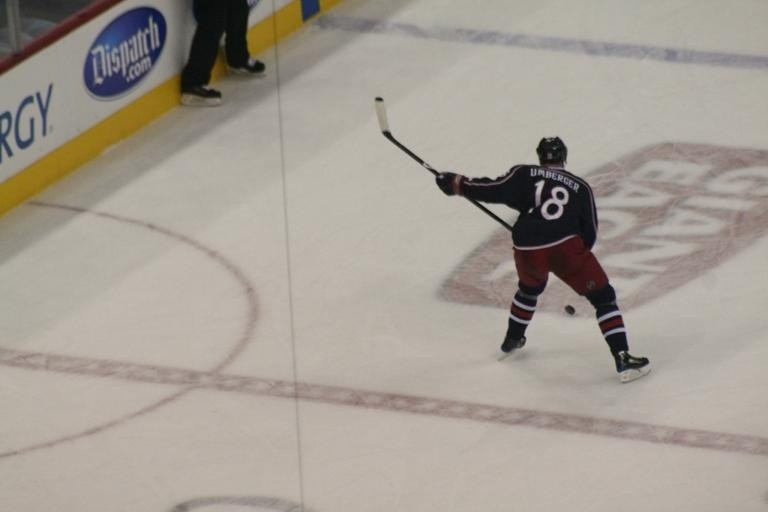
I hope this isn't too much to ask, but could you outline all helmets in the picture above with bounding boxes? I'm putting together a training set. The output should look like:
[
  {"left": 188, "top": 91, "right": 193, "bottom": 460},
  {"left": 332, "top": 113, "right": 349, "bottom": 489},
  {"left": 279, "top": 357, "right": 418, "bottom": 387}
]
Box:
[{"left": 536, "top": 136, "right": 568, "bottom": 167}]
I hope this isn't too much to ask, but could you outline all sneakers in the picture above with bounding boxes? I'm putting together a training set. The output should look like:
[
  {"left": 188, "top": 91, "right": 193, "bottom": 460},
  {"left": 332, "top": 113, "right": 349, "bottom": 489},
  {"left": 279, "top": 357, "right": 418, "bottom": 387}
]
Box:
[
  {"left": 613, "top": 347, "right": 649, "bottom": 373},
  {"left": 227, "top": 54, "right": 265, "bottom": 73},
  {"left": 181, "top": 82, "right": 221, "bottom": 98},
  {"left": 501, "top": 334, "right": 527, "bottom": 353}
]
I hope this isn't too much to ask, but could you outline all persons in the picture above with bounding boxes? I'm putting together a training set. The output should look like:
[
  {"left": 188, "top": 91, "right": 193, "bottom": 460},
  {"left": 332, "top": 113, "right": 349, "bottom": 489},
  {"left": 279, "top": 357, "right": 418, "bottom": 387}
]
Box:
[
  {"left": 435, "top": 134, "right": 651, "bottom": 374},
  {"left": 180, "top": 0, "right": 266, "bottom": 98}
]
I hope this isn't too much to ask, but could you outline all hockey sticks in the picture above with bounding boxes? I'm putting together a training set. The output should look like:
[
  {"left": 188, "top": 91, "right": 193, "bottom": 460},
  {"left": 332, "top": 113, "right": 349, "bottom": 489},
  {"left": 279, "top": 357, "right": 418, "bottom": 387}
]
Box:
[{"left": 374, "top": 97, "right": 515, "bottom": 230}]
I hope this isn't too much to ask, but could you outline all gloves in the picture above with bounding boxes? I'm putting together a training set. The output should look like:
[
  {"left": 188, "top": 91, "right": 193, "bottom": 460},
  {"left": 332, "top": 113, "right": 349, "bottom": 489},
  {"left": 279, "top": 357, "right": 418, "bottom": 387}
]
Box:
[{"left": 436, "top": 172, "right": 467, "bottom": 196}]
